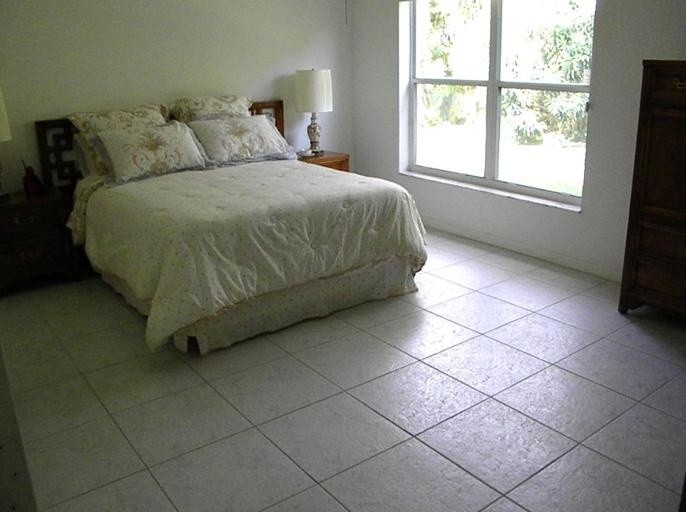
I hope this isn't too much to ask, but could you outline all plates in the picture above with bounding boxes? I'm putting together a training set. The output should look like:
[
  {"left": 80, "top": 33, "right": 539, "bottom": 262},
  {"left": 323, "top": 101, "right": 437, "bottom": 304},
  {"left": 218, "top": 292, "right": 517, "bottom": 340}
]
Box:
[{"left": 301, "top": 153, "right": 315, "bottom": 157}]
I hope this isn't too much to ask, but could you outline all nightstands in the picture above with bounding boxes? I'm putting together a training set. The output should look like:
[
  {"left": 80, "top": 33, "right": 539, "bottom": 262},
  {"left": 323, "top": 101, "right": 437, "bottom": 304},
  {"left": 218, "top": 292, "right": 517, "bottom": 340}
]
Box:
[
  {"left": 0, "top": 186, "right": 84, "bottom": 297},
  {"left": 296, "top": 148, "right": 353, "bottom": 174}
]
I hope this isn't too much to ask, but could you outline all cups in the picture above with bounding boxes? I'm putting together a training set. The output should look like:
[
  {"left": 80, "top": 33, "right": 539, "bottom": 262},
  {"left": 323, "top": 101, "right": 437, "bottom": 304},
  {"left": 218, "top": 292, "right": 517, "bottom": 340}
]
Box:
[{"left": 303, "top": 149, "right": 312, "bottom": 156}]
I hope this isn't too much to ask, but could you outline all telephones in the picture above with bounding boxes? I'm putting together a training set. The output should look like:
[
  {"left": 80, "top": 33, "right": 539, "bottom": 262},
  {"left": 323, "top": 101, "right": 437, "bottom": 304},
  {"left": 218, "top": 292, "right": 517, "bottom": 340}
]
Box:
[{"left": 21, "top": 160, "right": 45, "bottom": 194}]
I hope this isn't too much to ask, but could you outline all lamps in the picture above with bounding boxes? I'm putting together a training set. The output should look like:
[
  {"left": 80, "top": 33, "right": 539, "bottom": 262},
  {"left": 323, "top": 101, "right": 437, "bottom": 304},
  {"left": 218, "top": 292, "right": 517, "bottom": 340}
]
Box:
[{"left": 293, "top": 66, "right": 336, "bottom": 156}]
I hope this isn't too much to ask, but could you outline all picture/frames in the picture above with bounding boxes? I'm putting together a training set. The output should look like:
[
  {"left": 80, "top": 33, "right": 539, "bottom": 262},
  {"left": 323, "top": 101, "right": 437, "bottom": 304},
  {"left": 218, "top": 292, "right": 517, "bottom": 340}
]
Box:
[
  {"left": 30, "top": 114, "right": 83, "bottom": 182},
  {"left": 250, "top": 98, "right": 287, "bottom": 141}
]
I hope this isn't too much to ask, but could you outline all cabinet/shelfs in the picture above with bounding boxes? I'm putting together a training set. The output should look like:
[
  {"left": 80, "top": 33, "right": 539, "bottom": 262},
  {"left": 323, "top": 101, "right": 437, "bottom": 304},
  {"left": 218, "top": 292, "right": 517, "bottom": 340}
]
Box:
[{"left": 618, "top": 59, "right": 686, "bottom": 323}]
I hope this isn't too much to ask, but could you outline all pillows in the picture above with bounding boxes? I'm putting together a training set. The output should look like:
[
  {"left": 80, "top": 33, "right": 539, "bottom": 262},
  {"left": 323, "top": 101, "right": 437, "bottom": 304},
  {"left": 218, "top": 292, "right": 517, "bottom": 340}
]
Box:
[{"left": 71, "top": 98, "right": 301, "bottom": 189}]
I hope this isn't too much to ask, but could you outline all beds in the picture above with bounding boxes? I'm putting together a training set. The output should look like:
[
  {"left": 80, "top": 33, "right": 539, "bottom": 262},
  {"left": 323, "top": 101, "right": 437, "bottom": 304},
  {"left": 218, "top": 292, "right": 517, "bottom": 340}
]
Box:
[{"left": 66, "top": 89, "right": 430, "bottom": 364}]
